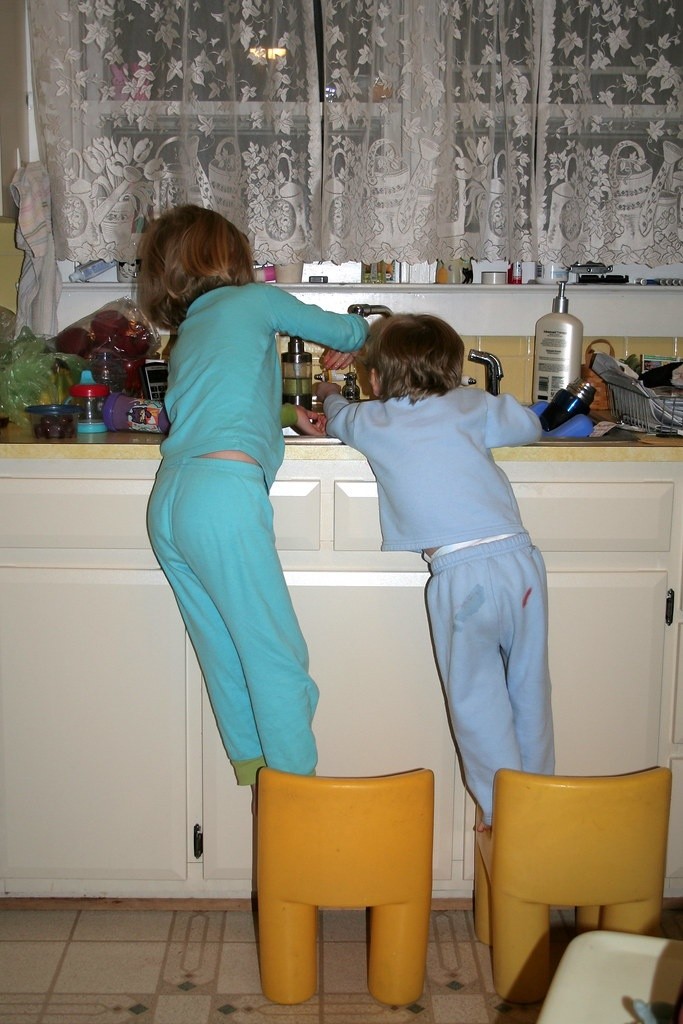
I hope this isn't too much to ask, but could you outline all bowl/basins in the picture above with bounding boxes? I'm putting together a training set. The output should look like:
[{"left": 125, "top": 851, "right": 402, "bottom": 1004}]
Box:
[{"left": 25, "top": 405, "right": 81, "bottom": 440}]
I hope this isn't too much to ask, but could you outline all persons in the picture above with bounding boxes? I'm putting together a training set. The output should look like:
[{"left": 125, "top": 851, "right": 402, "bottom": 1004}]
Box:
[
  {"left": 133, "top": 204, "right": 369, "bottom": 816},
  {"left": 314, "top": 314, "right": 555, "bottom": 831}
]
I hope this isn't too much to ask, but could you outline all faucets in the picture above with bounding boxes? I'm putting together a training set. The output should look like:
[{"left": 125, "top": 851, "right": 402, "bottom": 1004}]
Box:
[{"left": 346, "top": 303, "right": 393, "bottom": 318}]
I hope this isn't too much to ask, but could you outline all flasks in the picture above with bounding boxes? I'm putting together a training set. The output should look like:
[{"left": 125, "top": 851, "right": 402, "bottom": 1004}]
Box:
[{"left": 538, "top": 377, "right": 596, "bottom": 432}]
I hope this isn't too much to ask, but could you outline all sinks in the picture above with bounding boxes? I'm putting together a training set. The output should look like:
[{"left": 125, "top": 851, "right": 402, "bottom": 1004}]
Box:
[{"left": 281, "top": 411, "right": 330, "bottom": 436}]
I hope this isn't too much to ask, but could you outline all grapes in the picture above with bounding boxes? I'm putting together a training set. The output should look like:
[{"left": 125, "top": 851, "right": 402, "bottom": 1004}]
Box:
[{"left": 34, "top": 415, "right": 72, "bottom": 438}]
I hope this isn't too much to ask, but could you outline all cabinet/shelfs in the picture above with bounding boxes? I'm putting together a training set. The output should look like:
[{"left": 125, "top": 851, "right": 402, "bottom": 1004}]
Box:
[{"left": 1, "top": 445, "right": 683, "bottom": 915}]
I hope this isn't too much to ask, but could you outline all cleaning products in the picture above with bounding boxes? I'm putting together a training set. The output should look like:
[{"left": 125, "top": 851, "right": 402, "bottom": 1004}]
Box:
[{"left": 281, "top": 330, "right": 314, "bottom": 408}]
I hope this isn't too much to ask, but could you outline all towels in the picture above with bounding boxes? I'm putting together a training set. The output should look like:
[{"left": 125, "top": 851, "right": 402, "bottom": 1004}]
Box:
[{"left": 9, "top": 159, "right": 63, "bottom": 337}]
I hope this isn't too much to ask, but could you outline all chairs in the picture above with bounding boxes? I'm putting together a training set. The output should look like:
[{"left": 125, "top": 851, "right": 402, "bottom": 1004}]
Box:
[
  {"left": 474, "top": 765, "right": 674, "bottom": 1007},
  {"left": 252, "top": 766, "right": 435, "bottom": 1007}
]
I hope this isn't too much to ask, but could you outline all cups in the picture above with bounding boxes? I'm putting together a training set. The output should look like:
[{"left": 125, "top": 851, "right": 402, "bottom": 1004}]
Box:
[
  {"left": 117, "top": 259, "right": 140, "bottom": 283},
  {"left": 65, "top": 371, "right": 112, "bottom": 432}
]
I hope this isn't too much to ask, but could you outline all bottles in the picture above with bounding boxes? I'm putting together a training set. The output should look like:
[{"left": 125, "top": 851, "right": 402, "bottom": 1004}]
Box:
[{"left": 69, "top": 260, "right": 117, "bottom": 282}]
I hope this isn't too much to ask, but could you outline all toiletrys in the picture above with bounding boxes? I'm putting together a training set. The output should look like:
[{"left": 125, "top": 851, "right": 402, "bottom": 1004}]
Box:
[{"left": 529, "top": 279, "right": 585, "bottom": 404}]
